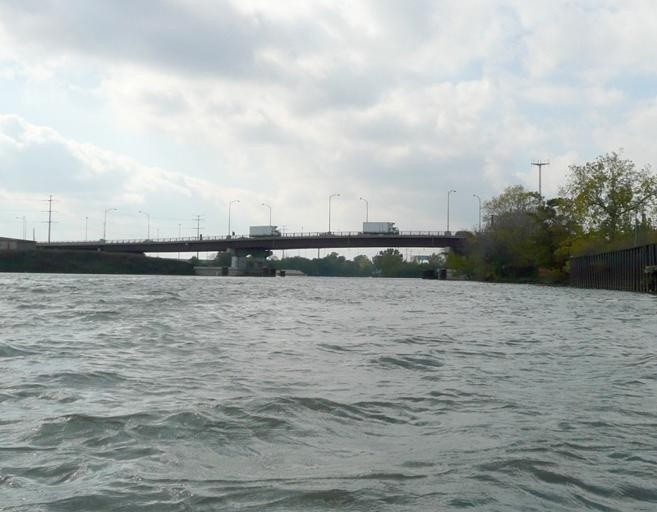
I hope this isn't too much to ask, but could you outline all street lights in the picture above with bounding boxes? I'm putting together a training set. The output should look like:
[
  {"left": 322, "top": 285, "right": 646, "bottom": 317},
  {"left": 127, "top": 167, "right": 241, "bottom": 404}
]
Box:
[
  {"left": 472, "top": 193, "right": 481, "bottom": 235},
  {"left": 260, "top": 203, "right": 271, "bottom": 225},
  {"left": 228, "top": 200, "right": 240, "bottom": 236},
  {"left": 447, "top": 189, "right": 456, "bottom": 231},
  {"left": 360, "top": 197, "right": 368, "bottom": 221},
  {"left": 138, "top": 210, "right": 149, "bottom": 238},
  {"left": 328, "top": 193, "right": 340, "bottom": 234},
  {"left": 85, "top": 216, "right": 88, "bottom": 240},
  {"left": 15, "top": 217, "right": 27, "bottom": 240},
  {"left": 104, "top": 207, "right": 116, "bottom": 239}
]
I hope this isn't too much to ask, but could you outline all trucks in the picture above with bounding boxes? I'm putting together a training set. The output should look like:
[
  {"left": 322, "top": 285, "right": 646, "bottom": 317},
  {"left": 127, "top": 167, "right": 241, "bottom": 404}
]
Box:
[
  {"left": 248, "top": 226, "right": 280, "bottom": 237},
  {"left": 362, "top": 221, "right": 399, "bottom": 235}
]
